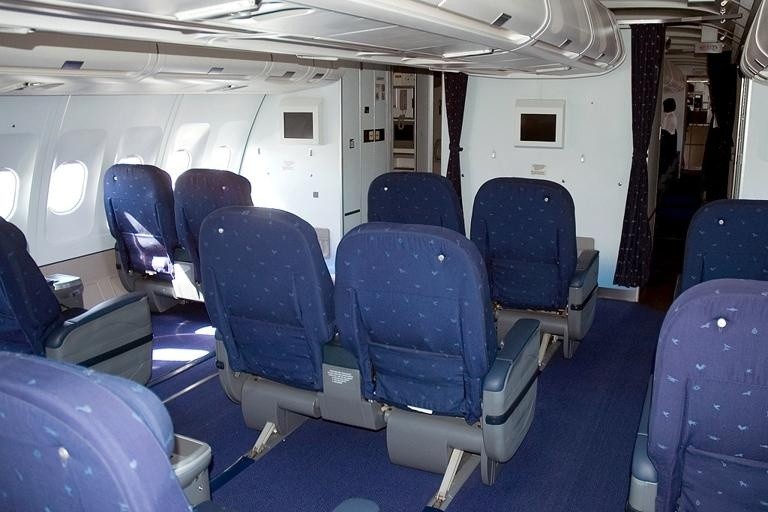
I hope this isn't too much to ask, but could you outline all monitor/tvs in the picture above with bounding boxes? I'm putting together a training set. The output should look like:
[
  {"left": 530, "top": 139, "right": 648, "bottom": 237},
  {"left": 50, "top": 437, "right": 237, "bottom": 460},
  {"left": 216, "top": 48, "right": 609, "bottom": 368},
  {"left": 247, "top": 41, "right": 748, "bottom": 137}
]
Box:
[
  {"left": 513, "top": 99, "right": 564, "bottom": 148},
  {"left": 280, "top": 97, "right": 320, "bottom": 145}
]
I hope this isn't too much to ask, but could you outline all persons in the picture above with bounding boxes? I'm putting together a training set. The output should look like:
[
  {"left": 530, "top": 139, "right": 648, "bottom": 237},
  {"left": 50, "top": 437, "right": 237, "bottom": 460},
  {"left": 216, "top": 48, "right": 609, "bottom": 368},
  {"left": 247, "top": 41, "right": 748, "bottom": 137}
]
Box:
[{"left": 659, "top": 98, "right": 678, "bottom": 176}]
